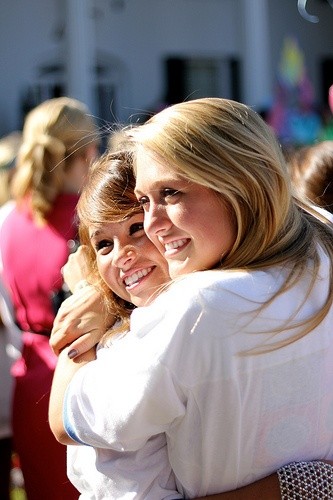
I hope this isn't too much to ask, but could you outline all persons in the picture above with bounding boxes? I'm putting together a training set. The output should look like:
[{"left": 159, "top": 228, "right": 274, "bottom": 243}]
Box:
[{"left": 0, "top": 93, "right": 333, "bottom": 499}]
